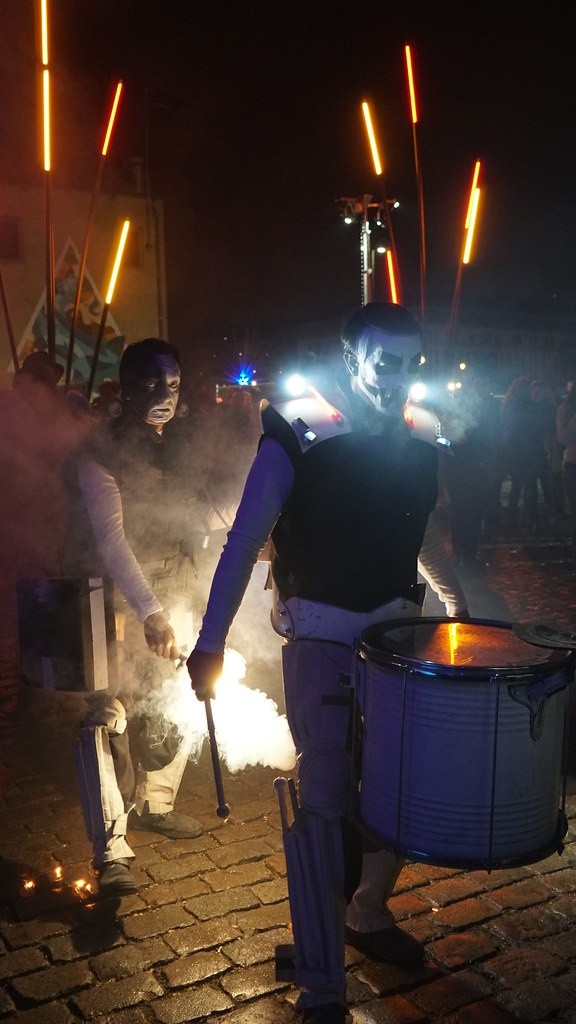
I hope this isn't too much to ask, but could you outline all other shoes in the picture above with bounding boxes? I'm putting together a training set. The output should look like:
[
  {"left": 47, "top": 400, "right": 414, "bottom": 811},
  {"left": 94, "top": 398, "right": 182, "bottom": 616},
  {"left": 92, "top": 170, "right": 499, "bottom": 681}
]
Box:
[
  {"left": 344, "top": 925, "right": 425, "bottom": 967},
  {"left": 302, "top": 1002, "right": 347, "bottom": 1024}
]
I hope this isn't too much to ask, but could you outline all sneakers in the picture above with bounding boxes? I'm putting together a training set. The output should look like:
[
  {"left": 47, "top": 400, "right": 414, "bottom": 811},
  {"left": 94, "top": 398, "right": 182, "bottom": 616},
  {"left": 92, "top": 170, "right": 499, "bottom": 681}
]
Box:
[
  {"left": 130, "top": 810, "right": 203, "bottom": 838},
  {"left": 97, "top": 862, "right": 139, "bottom": 897}
]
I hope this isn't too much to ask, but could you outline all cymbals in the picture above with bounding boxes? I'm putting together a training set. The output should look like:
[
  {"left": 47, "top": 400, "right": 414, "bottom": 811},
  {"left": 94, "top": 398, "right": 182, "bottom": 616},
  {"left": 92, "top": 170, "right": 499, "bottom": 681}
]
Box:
[{"left": 514, "top": 619, "right": 576, "bottom": 651}]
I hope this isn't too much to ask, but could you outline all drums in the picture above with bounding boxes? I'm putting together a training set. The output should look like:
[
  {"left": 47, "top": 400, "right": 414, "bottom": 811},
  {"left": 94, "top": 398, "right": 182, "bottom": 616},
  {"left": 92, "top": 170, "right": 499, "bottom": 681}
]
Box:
[
  {"left": 358, "top": 618, "right": 576, "bottom": 872},
  {"left": 14, "top": 573, "right": 88, "bottom": 695}
]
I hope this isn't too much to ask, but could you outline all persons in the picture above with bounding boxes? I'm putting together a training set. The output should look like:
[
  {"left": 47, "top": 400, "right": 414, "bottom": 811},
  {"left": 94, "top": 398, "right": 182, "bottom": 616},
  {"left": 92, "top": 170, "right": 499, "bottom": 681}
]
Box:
[
  {"left": 57, "top": 339, "right": 221, "bottom": 896},
  {"left": 185, "top": 302, "right": 474, "bottom": 1024},
  {"left": 0, "top": 351, "right": 576, "bottom": 632}
]
